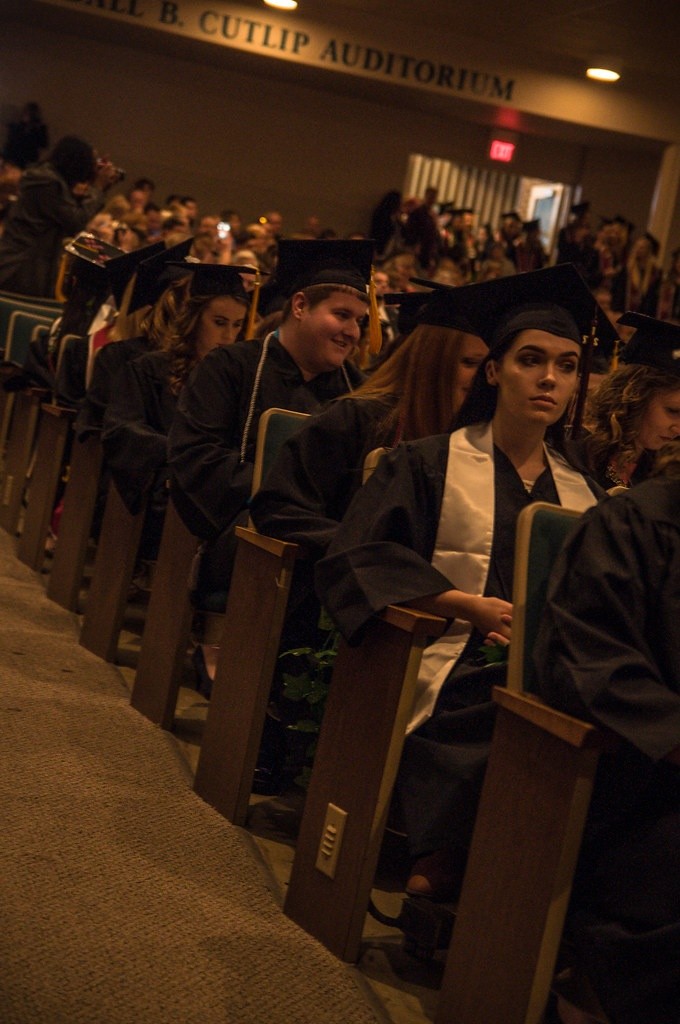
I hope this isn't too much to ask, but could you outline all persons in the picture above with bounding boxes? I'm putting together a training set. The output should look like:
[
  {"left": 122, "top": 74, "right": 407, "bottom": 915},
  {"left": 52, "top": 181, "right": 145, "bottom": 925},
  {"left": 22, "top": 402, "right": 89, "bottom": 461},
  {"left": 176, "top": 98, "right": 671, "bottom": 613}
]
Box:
[{"left": 0, "top": 101, "right": 680, "bottom": 1024}]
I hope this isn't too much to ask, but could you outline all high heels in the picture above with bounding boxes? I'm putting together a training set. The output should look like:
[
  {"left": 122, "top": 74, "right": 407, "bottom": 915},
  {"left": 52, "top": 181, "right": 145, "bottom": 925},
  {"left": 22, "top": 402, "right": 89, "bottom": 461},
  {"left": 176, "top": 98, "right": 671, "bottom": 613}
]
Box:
[{"left": 192, "top": 644, "right": 214, "bottom": 701}]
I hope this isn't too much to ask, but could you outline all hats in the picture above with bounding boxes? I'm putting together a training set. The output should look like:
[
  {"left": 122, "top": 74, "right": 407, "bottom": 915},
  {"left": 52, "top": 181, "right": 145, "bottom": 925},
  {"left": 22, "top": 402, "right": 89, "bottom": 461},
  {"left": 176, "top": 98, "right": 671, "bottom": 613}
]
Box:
[
  {"left": 104, "top": 240, "right": 167, "bottom": 312},
  {"left": 383, "top": 292, "right": 431, "bottom": 329},
  {"left": 166, "top": 261, "right": 271, "bottom": 342},
  {"left": 117, "top": 237, "right": 196, "bottom": 335},
  {"left": 274, "top": 239, "right": 383, "bottom": 354},
  {"left": 448, "top": 262, "right": 620, "bottom": 441},
  {"left": 616, "top": 310, "right": 680, "bottom": 375},
  {"left": 408, "top": 277, "right": 479, "bottom": 335}
]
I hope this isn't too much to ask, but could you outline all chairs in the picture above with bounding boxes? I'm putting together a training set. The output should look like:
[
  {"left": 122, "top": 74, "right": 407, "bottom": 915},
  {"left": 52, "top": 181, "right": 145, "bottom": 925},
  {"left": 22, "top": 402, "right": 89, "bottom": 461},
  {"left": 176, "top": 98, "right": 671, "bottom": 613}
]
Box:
[{"left": 0, "top": 290, "right": 630, "bottom": 1024}]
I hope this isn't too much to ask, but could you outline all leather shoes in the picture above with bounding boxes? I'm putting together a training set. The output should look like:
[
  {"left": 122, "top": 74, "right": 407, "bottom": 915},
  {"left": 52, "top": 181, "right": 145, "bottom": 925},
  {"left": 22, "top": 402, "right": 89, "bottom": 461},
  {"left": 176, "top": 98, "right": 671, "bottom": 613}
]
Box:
[{"left": 406, "top": 849, "right": 460, "bottom": 898}]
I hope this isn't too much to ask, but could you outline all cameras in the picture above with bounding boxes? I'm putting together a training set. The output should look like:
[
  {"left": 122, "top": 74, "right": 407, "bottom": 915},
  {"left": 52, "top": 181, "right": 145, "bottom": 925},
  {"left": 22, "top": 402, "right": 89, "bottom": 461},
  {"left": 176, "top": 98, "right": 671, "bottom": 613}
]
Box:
[{"left": 96, "top": 163, "right": 125, "bottom": 183}]
